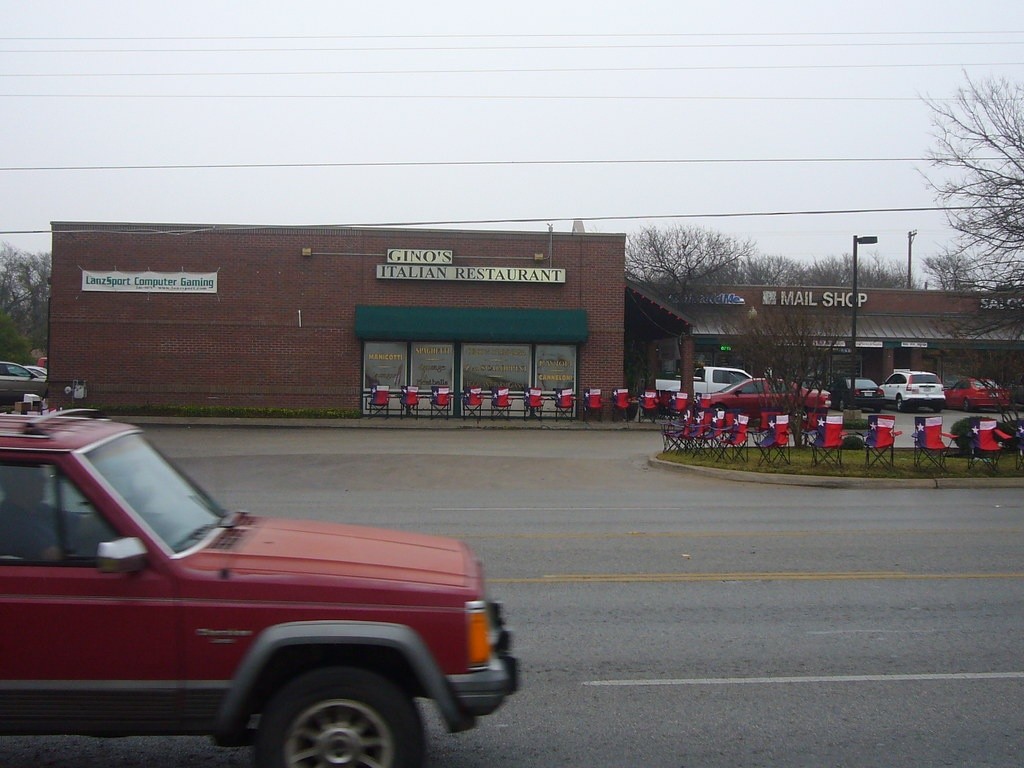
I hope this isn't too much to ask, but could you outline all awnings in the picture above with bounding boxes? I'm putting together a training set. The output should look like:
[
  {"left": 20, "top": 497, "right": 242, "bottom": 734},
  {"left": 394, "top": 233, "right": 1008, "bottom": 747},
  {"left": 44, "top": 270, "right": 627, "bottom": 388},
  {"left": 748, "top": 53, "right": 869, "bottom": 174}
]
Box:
[{"left": 353, "top": 306, "right": 589, "bottom": 343}]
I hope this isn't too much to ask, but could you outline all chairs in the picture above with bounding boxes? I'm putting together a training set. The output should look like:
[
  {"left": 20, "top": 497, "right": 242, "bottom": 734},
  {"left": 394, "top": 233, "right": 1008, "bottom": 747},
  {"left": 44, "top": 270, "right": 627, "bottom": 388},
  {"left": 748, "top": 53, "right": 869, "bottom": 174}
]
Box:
[
  {"left": 757, "top": 412, "right": 794, "bottom": 465},
  {"left": 365, "top": 383, "right": 392, "bottom": 421},
  {"left": 461, "top": 385, "right": 484, "bottom": 423},
  {"left": 911, "top": 412, "right": 957, "bottom": 471},
  {"left": 398, "top": 383, "right": 421, "bottom": 421},
  {"left": 1014, "top": 425, "right": 1024, "bottom": 471},
  {"left": 637, "top": 389, "right": 662, "bottom": 424},
  {"left": 427, "top": 383, "right": 454, "bottom": 421},
  {"left": 665, "top": 392, "right": 689, "bottom": 421},
  {"left": 611, "top": 387, "right": 633, "bottom": 423},
  {"left": 582, "top": 385, "right": 606, "bottom": 421},
  {"left": 689, "top": 391, "right": 715, "bottom": 417},
  {"left": 661, "top": 410, "right": 750, "bottom": 464},
  {"left": 857, "top": 412, "right": 904, "bottom": 467},
  {"left": 798, "top": 410, "right": 828, "bottom": 445},
  {"left": 551, "top": 387, "right": 577, "bottom": 422},
  {"left": 968, "top": 417, "right": 1012, "bottom": 469},
  {"left": 808, "top": 412, "right": 846, "bottom": 468},
  {"left": 750, "top": 409, "right": 784, "bottom": 448},
  {"left": 489, "top": 387, "right": 513, "bottom": 421},
  {"left": 521, "top": 386, "right": 546, "bottom": 423}
]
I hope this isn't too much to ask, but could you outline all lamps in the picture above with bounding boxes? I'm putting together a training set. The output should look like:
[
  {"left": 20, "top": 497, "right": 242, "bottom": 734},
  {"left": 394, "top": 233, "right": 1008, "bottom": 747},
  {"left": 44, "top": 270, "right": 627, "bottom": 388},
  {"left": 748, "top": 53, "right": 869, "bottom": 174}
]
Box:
[
  {"left": 301, "top": 248, "right": 312, "bottom": 257},
  {"left": 534, "top": 254, "right": 544, "bottom": 261},
  {"left": 747, "top": 305, "right": 758, "bottom": 320}
]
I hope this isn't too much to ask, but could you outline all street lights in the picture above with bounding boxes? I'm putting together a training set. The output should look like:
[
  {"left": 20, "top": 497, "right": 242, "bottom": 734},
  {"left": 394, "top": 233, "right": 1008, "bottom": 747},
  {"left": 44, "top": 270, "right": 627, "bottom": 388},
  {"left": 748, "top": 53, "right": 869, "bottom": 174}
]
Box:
[{"left": 848, "top": 234, "right": 878, "bottom": 409}]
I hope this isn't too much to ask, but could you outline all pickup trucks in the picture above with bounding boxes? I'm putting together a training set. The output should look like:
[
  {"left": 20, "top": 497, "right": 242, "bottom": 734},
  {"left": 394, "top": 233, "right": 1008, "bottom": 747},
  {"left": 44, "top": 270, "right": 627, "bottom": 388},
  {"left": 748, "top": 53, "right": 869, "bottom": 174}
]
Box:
[
  {"left": 652, "top": 363, "right": 756, "bottom": 413},
  {"left": 0, "top": 402, "right": 523, "bottom": 768}
]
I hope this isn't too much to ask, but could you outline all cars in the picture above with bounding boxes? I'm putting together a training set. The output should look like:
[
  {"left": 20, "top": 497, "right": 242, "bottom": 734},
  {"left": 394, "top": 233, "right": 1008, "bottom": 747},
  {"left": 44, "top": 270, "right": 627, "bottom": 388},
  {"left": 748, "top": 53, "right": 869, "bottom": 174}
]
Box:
[
  {"left": 0, "top": 360, "right": 49, "bottom": 407},
  {"left": 827, "top": 376, "right": 886, "bottom": 412},
  {"left": 707, "top": 376, "right": 832, "bottom": 420},
  {"left": 945, "top": 377, "right": 1010, "bottom": 412}
]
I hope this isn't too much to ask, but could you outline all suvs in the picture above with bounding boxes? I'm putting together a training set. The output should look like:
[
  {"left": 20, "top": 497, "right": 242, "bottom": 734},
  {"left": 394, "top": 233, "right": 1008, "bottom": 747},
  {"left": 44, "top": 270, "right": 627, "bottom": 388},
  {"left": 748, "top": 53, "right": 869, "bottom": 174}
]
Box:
[{"left": 877, "top": 372, "right": 947, "bottom": 412}]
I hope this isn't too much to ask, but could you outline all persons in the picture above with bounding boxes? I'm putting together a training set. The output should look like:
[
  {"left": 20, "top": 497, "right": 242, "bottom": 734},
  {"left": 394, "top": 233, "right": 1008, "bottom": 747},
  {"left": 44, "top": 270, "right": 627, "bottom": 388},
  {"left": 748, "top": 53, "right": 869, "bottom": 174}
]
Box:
[{"left": 0, "top": 466, "right": 110, "bottom": 560}]
